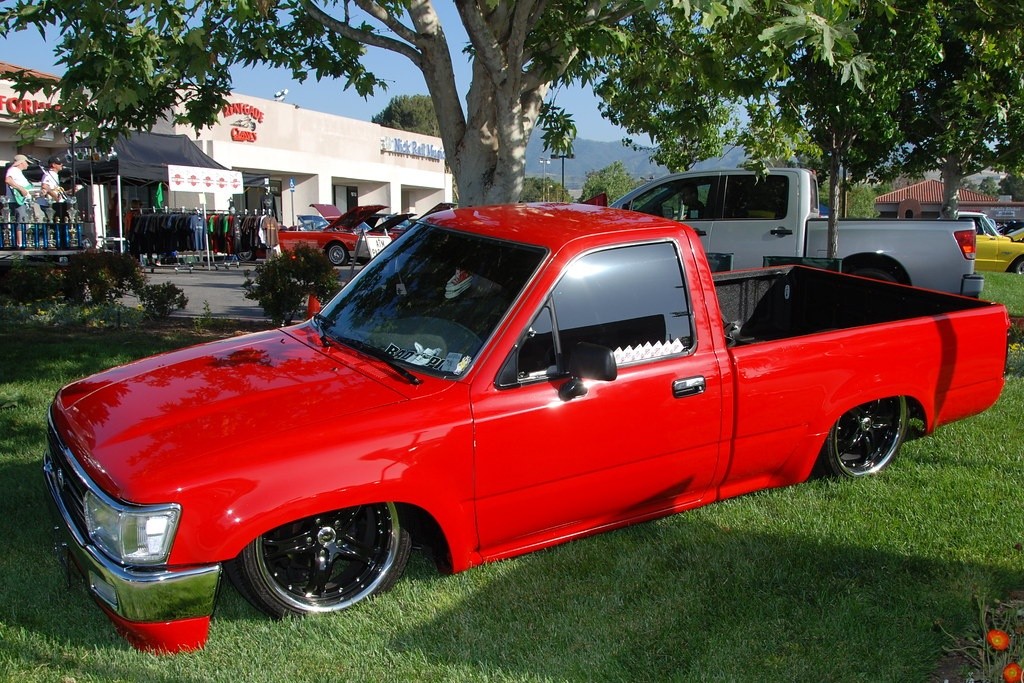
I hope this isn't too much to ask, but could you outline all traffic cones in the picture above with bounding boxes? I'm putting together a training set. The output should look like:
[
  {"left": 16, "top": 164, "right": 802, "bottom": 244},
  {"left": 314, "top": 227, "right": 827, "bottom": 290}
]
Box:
[{"left": 304, "top": 293, "right": 320, "bottom": 321}]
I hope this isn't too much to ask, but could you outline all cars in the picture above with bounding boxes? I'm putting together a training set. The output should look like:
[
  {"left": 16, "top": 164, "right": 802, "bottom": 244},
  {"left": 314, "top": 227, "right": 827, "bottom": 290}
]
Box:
[{"left": 275, "top": 201, "right": 457, "bottom": 265}]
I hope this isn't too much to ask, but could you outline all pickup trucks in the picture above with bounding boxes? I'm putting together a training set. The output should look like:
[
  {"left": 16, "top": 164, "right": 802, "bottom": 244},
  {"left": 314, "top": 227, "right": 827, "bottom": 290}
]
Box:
[
  {"left": 606, "top": 168, "right": 982, "bottom": 301},
  {"left": 41, "top": 199, "right": 1010, "bottom": 652}
]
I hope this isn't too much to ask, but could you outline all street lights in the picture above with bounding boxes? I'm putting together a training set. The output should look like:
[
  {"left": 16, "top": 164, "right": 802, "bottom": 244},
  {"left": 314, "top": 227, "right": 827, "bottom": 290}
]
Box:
[
  {"left": 550, "top": 153, "right": 574, "bottom": 201},
  {"left": 539, "top": 156, "right": 550, "bottom": 201}
]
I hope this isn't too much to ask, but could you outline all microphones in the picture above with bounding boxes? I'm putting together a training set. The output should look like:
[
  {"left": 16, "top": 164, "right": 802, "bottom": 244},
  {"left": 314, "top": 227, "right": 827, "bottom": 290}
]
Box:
[
  {"left": 63, "top": 165, "right": 72, "bottom": 169},
  {"left": 28, "top": 155, "right": 41, "bottom": 162}
]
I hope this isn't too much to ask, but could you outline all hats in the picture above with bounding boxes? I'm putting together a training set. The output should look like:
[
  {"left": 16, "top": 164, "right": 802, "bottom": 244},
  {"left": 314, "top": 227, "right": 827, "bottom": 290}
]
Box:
[
  {"left": 14, "top": 154, "right": 34, "bottom": 164},
  {"left": 48, "top": 156, "right": 63, "bottom": 165}
]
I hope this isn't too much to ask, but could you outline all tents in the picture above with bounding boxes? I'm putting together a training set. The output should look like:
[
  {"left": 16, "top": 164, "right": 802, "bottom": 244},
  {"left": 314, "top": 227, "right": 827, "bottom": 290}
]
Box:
[{"left": 47, "top": 128, "right": 280, "bottom": 271}]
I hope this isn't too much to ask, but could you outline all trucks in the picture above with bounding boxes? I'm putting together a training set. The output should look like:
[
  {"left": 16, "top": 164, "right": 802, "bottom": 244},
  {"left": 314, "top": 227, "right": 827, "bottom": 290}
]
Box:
[{"left": 954, "top": 211, "right": 1024, "bottom": 275}]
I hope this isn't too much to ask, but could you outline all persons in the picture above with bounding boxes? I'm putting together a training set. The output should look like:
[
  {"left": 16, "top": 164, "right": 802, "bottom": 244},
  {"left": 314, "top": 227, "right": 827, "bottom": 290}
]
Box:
[
  {"left": 108, "top": 194, "right": 142, "bottom": 236},
  {"left": 681, "top": 183, "right": 705, "bottom": 219},
  {"left": 41, "top": 157, "right": 74, "bottom": 250},
  {"left": 4, "top": 153, "right": 34, "bottom": 249}
]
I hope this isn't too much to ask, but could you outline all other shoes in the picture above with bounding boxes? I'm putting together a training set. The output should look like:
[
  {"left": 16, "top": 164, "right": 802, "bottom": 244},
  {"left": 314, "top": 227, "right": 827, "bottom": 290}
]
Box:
[{"left": 155, "top": 261, "right": 161, "bottom": 266}]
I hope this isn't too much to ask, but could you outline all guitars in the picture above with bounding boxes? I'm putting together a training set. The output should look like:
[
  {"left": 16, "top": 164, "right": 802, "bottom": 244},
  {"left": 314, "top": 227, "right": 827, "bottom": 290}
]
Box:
[
  {"left": 47, "top": 184, "right": 83, "bottom": 204},
  {"left": 13, "top": 184, "right": 41, "bottom": 206}
]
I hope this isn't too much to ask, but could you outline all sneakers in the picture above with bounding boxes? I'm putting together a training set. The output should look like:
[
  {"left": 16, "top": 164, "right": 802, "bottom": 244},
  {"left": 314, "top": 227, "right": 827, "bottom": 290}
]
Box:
[
  {"left": 445, "top": 282, "right": 471, "bottom": 299},
  {"left": 446, "top": 270, "right": 474, "bottom": 291}
]
